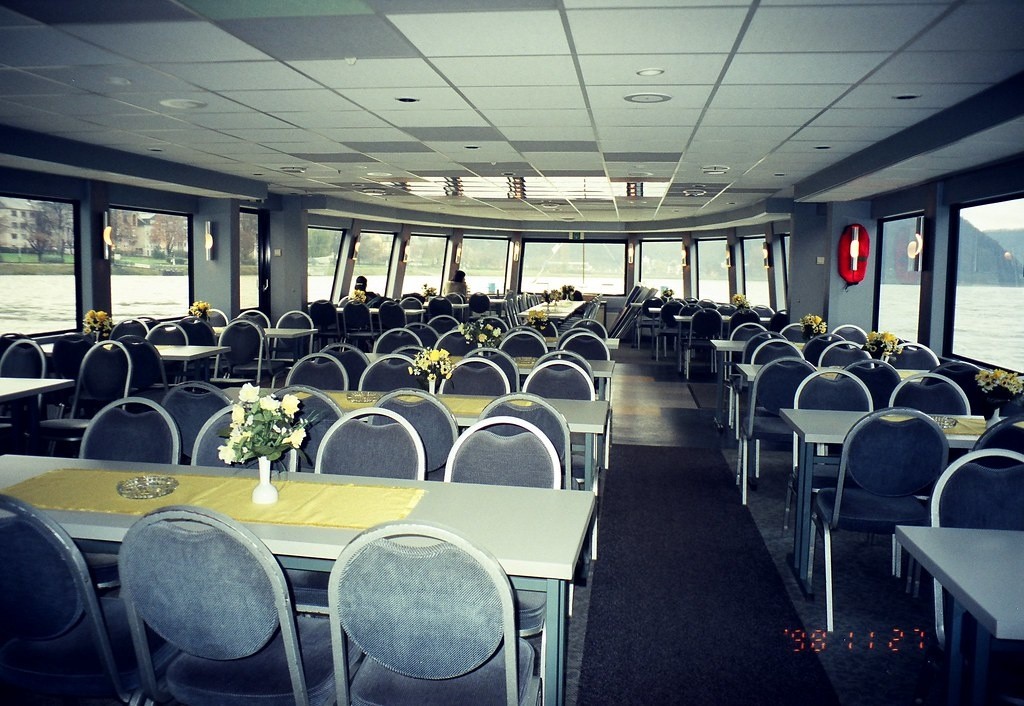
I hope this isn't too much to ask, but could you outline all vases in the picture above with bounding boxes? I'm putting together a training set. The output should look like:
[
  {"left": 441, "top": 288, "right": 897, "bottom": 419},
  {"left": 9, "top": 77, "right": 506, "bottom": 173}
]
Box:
[
  {"left": 477, "top": 343, "right": 484, "bottom": 357},
  {"left": 94, "top": 331, "right": 100, "bottom": 343},
  {"left": 428, "top": 379, "right": 436, "bottom": 395},
  {"left": 987, "top": 407, "right": 1001, "bottom": 430},
  {"left": 252, "top": 456, "right": 278, "bottom": 504}
]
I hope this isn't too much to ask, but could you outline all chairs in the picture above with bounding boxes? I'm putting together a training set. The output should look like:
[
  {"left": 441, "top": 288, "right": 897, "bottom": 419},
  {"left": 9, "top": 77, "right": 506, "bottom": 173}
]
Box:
[{"left": 1, "top": 287, "right": 1023, "bottom": 706}]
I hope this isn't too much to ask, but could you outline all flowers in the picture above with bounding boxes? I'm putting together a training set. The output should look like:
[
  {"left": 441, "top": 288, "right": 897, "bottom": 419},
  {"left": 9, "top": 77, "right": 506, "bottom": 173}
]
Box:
[
  {"left": 347, "top": 289, "right": 367, "bottom": 304},
  {"left": 528, "top": 311, "right": 548, "bottom": 331},
  {"left": 799, "top": 313, "right": 827, "bottom": 334},
  {"left": 82, "top": 310, "right": 112, "bottom": 338},
  {"left": 541, "top": 289, "right": 561, "bottom": 303},
  {"left": 189, "top": 301, "right": 211, "bottom": 322},
  {"left": 422, "top": 284, "right": 436, "bottom": 298},
  {"left": 562, "top": 285, "right": 575, "bottom": 295},
  {"left": 217, "top": 382, "right": 319, "bottom": 465},
  {"left": 458, "top": 319, "right": 503, "bottom": 345},
  {"left": 864, "top": 330, "right": 904, "bottom": 357},
  {"left": 732, "top": 294, "right": 750, "bottom": 308},
  {"left": 662, "top": 288, "right": 675, "bottom": 298},
  {"left": 408, "top": 344, "right": 453, "bottom": 381},
  {"left": 975, "top": 368, "right": 1024, "bottom": 395}
]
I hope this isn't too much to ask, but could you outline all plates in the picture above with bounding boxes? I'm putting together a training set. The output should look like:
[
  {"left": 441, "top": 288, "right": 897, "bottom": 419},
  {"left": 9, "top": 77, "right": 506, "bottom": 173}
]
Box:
[
  {"left": 929, "top": 416, "right": 957, "bottom": 428},
  {"left": 829, "top": 366, "right": 845, "bottom": 370},
  {"left": 513, "top": 357, "right": 539, "bottom": 364},
  {"left": 117, "top": 476, "right": 178, "bottom": 499},
  {"left": 347, "top": 391, "right": 379, "bottom": 403}
]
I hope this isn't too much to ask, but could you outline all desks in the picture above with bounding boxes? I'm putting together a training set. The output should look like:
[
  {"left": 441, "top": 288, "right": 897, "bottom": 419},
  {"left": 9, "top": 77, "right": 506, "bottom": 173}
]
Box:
[
  {"left": 648, "top": 308, "right": 661, "bottom": 360},
  {"left": 0, "top": 377, "right": 74, "bottom": 403},
  {"left": 542, "top": 337, "right": 620, "bottom": 349},
  {"left": 38, "top": 343, "right": 231, "bottom": 382},
  {"left": 163, "top": 327, "right": 318, "bottom": 364},
  {"left": 364, "top": 352, "right": 615, "bottom": 401},
  {"left": 517, "top": 300, "right": 587, "bottom": 320},
  {"left": 423, "top": 301, "right": 469, "bottom": 319},
  {"left": 735, "top": 364, "right": 921, "bottom": 490},
  {"left": 709, "top": 339, "right": 865, "bottom": 435},
  {"left": 630, "top": 303, "right": 644, "bottom": 351},
  {"left": 895, "top": 524, "right": 1023, "bottom": 706},
  {"left": 0, "top": 454, "right": 597, "bottom": 706},
  {"left": 779, "top": 408, "right": 987, "bottom": 601},
  {"left": 674, "top": 315, "right": 772, "bottom": 376},
  {"left": 220, "top": 387, "right": 609, "bottom": 490},
  {"left": 489, "top": 298, "right": 507, "bottom": 305},
  {"left": 307, "top": 305, "right": 426, "bottom": 330}
]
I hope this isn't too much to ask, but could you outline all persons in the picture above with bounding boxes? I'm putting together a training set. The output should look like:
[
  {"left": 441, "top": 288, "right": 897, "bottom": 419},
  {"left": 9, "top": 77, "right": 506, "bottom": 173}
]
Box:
[
  {"left": 355, "top": 275, "right": 381, "bottom": 307},
  {"left": 443, "top": 271, "right": 467, "bottom": 297}
]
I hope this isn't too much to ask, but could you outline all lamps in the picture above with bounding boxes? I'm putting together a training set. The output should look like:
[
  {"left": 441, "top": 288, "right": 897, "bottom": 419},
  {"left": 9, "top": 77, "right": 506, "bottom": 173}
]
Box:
[
  {"left": 909, "top": 215, "right": 923, "bottom": 273},
  {"left": 104, "top": 211, "right": 769, "bottom": 269}
]
